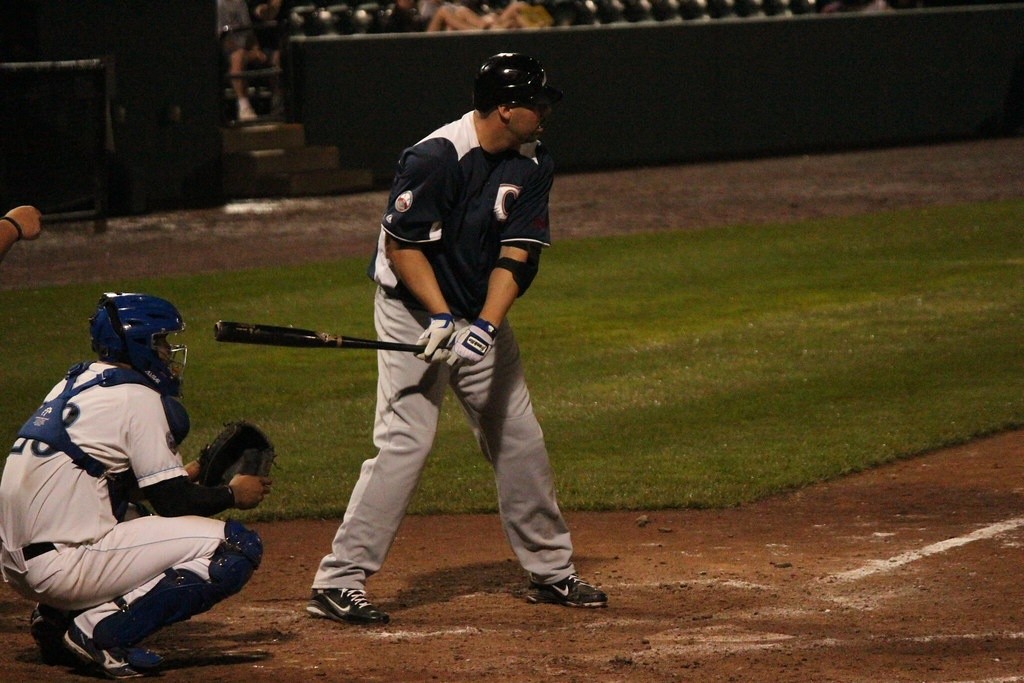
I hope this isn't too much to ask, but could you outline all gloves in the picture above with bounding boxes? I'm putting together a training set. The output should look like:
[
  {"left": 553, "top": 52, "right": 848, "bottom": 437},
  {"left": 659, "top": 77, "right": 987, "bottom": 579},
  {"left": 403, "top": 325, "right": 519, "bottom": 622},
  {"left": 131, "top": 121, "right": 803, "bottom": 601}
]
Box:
[
  {"left": 413, "top": 313, "right": 451, "bottom": 363},
  {"left": 445, "top": 318, "right": 497, "bottom": 368}
]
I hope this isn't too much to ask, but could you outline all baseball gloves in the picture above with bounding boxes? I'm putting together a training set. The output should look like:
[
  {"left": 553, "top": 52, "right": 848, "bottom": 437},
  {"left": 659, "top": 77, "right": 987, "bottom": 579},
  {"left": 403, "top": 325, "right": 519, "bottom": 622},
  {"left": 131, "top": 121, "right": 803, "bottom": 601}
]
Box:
[{"left": 198, "top": 420, "right": 276, "bottom": 487}]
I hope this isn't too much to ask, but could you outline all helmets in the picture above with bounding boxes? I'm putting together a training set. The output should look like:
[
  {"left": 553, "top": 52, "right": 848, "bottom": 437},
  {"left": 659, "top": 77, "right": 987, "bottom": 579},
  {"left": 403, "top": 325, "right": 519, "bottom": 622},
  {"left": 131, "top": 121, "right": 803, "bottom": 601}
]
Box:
[
  {"left": 87, "top": 292, "right": 187, "bottom": 396},
  {"left": 473, "top": 53, "right": 563, "bottom": 113}
]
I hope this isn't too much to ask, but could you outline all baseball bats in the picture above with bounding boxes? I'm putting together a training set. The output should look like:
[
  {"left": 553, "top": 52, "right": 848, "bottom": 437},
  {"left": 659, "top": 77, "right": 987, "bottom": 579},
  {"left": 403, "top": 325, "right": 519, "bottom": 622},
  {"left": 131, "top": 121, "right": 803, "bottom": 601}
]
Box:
[{"left": 213, "top": 319, "right": 427, "bottom": 355}]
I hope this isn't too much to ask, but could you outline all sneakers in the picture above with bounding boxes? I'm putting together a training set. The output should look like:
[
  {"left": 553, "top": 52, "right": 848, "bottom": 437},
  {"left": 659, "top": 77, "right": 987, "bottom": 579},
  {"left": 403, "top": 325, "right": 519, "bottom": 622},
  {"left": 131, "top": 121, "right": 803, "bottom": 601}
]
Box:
[
  {"left": 527, "top": 574, "right": 608, "bottom": 607},
  {"left": 30, "top": 606, "right": 70, "bottom": 665},
  {"left": 62, "top": 623, "right": 148, "bottom": 678},
  {"left": 306, "top": 587, "right": 390, "bottom": 625}
]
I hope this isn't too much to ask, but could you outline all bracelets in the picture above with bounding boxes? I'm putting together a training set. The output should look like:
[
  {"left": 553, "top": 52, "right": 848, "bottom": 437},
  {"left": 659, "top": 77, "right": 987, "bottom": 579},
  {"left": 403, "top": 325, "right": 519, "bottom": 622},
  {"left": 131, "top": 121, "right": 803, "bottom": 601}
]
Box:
[{"left": 0, "top": 216, "right": 23, "bottom": 242}]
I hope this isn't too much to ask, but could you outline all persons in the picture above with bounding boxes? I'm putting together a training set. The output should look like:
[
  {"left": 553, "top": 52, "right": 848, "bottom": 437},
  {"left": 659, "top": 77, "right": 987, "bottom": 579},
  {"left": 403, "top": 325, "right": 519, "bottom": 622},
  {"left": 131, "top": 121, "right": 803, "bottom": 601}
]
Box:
[
  {"left": 216, "top": 0, "right": 286, "bottom": 127},
  {"left": 386, "top": 0, "right": 570, "bottom": 33},
  {"left": 1, "top": 204, "right": 41, "bottom": 260},
  {"left": 301, "top": 51, "right": 610, "bottom": 629},
  {"left": 0, "top": 292, "right": 275, "bottom": 679}
]
